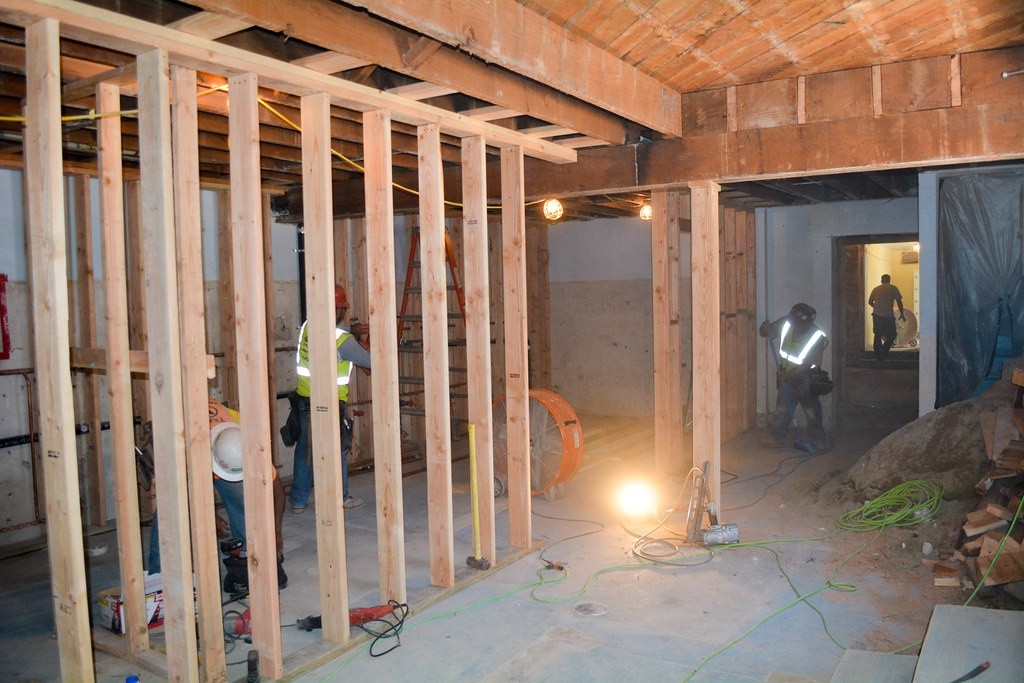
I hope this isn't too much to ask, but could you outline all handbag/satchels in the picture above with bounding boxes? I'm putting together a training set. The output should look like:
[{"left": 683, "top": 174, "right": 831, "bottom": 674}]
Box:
[
  {"left": 280, "top": 392, "right": 300, "bottom": 447},
  {"left": 341, "top": 413, "right": 355, "bottom": 456},
  {"left": 810, "top": 370, "right": 834, "bottom": 395}
]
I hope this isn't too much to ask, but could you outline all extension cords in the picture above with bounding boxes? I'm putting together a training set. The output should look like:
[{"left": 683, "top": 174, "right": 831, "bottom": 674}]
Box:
[{"left": 546, "top": 560, "right": 565, "bottom": 571}]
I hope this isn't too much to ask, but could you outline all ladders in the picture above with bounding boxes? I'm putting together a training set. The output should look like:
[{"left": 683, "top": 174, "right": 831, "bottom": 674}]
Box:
[{"left": 397, "top": 226, "right": 468, "bottom": 423}]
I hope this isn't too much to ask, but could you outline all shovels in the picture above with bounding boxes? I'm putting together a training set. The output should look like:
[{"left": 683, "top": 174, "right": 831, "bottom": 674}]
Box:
[{"left": 766, "top": 324, "right": 819, "bottom": 455}]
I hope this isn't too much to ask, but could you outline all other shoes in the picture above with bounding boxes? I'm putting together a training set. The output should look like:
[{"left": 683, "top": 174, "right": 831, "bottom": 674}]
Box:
[
  {"left": 761, "top": 436, "right": 784, "bottom": 447},
  {"left": 813, "top": 438, "right": 825, "bottom": 449}
]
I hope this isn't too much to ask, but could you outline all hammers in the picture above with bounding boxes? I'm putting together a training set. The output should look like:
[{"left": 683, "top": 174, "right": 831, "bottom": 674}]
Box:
[{"left": 465, "top": 423, "right": 491, "bottom": 571}]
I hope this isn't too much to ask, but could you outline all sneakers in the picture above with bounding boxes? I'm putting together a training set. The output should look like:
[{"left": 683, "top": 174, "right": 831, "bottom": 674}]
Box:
[
  {"left": 343, "top": 496, "right": 365, "bottom": 511},
  {"left": 292, "top": 499, "right": 309, "bottom": 514}
]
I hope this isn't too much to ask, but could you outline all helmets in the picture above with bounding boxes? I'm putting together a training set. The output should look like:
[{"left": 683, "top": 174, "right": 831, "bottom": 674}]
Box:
[
  {"left": 210, "top": 422, "right": 243, "bottom": 482},
  {"left": 789, "top": 303, "right": 817, "bottom": 333},
  {"left": 335, "top": 283, "right": 348, "bottom": 308}
]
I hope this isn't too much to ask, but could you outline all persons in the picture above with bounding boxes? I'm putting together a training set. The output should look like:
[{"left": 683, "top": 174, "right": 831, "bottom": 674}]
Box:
[
  {"left": 147, "top": 397, "right": 286, "bottom": 575},
  {"left": 289, "top": 284, "right": 371, "bottom": 513},
  {"left": 868, "top": 274, "right": 906, "bottom": 356},
  {"left": 760, "top": 304, "right": 832, "bottom": 451}
]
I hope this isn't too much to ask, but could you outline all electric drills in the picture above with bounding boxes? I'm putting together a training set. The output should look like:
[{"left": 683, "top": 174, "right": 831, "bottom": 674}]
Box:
[{"left": 280, "top": 600, "right": 405, "bottom": 633}]
